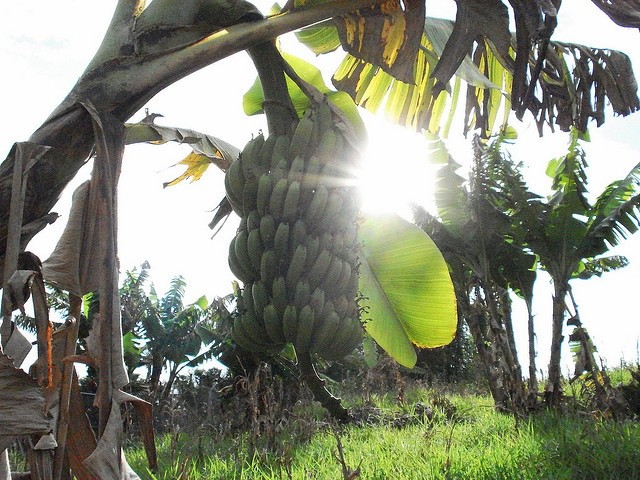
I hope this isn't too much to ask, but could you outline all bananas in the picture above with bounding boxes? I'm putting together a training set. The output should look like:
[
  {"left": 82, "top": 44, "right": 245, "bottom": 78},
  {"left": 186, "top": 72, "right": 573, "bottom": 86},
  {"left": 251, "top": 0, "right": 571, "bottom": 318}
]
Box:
[{"left": 226, "top": 99, "right": 364, "bottom": 359}]
